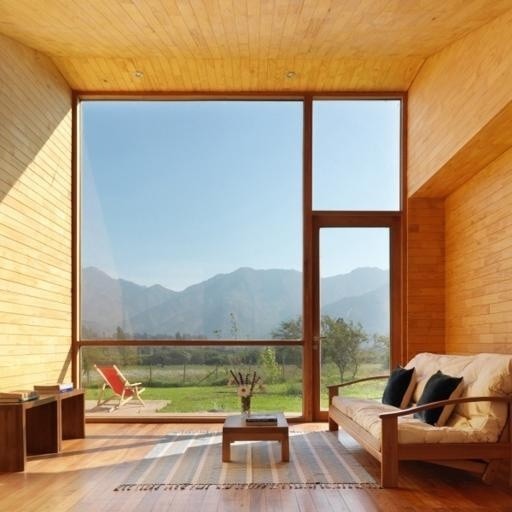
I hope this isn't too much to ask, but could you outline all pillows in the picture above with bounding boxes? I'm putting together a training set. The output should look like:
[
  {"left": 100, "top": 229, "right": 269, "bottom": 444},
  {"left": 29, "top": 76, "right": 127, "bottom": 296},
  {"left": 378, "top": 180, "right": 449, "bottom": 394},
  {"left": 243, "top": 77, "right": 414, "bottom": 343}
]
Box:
[{"left": 380, "top": 364, "right": 466, "bottom": 428}]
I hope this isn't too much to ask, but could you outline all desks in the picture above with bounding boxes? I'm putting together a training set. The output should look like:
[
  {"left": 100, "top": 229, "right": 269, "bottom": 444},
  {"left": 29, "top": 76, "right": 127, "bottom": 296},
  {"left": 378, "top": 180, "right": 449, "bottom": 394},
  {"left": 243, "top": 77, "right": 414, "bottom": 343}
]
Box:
[{"left": 0, "top": 389, "right": 85, "bottom": 472}]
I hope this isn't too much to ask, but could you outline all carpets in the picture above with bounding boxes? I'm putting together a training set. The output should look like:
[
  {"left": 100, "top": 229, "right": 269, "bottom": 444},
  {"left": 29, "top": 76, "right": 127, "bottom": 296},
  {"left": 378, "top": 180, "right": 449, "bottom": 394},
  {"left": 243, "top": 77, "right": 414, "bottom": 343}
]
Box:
[{"left": 111, "top": 429, "right": 380, "bottom": 490}]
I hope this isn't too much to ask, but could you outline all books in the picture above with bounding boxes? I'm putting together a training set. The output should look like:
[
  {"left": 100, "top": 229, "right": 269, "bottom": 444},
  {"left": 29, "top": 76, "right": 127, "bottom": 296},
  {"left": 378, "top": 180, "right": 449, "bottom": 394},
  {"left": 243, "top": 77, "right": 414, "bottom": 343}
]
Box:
[
  {"left": 245, "top": 415, "right": 277, "bottom": 426},
  {"left": 0, "top": 381, "right": 73, "bottom": 403}
]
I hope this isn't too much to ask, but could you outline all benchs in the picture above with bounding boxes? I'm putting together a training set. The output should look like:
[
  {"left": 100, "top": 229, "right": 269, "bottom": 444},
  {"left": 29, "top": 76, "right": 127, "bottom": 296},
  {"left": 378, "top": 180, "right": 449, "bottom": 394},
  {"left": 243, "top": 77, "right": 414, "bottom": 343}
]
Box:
[{"left": 324, "top": 351, "right": 512, "bottom": 489}]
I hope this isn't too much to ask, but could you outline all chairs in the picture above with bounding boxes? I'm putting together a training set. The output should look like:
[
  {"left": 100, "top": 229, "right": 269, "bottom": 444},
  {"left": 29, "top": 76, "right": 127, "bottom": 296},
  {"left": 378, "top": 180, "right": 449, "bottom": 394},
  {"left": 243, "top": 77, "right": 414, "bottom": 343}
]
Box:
[{"left": 92, "top": 364, "right": 147, "bottom": 412}]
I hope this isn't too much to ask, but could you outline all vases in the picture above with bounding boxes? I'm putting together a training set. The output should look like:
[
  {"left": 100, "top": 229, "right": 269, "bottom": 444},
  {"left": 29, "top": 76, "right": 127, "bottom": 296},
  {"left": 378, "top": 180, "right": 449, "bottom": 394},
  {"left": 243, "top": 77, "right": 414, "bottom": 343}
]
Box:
[{"left": 241, "top": 397, "right": 250, "bottom": 413}]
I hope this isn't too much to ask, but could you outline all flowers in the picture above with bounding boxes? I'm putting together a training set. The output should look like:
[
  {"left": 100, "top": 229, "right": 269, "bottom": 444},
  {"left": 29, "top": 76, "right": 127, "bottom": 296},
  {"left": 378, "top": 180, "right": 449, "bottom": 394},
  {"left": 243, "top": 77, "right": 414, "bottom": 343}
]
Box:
[{"left": 226, "top": 368, "right": 263, "bottom": 398}]
